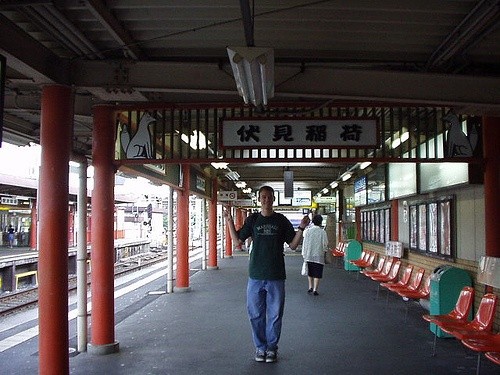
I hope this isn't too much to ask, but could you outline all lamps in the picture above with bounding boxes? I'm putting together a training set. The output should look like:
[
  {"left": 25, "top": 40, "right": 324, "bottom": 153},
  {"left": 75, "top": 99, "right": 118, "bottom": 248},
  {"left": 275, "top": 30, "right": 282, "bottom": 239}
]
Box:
[
  {"left": 226, "top": 46, "right": 274, "bottom": 107},
  {"left": 175, "top": 129, "right": 254, "bottom": 197},
  {"left": 315, "top": 130, "right": 409, "bottom": 196}
]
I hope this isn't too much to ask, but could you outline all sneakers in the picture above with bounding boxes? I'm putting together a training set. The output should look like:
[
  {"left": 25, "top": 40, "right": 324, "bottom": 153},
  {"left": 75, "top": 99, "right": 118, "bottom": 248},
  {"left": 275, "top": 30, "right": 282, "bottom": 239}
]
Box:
[
  {"left": 266, "top": 352, "right": 276, "bottom": 362},
  {"left": 254, "top": 350, "right": 265, "bottom": 361}
]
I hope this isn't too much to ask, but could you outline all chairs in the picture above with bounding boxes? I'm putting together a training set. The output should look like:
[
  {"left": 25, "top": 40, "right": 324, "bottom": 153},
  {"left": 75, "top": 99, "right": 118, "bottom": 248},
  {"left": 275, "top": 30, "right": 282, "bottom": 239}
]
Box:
[{"left": 330, "top": 242, "right": 500, "bottom": 375}]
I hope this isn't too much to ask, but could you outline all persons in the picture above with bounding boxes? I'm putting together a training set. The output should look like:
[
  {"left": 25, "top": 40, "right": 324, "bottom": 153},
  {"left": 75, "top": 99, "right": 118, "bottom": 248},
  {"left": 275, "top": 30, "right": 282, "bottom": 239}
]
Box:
[
  {"left": 302, "top": 215, "right": 328, "bottom": 295},
  {"left": 8, "top": 224, "right": 14, "bottom": 248},
  {"left": 224, "top": 186, "right": 311, "bottom": 362}
]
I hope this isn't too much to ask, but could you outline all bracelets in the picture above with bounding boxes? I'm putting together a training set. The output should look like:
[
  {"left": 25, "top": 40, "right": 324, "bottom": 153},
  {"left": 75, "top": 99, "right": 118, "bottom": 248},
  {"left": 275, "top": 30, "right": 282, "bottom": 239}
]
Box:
[{"left": 298, "top": 226, "right": 304, "bottom": 231}]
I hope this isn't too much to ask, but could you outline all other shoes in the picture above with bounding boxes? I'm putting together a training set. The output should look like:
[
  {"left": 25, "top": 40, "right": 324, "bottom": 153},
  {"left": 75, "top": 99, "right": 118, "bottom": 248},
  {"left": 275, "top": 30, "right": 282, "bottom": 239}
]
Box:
[
  {"left": 313, "top": 291, "right": 318, "bottom": 295},
  {"left": 308, "top": 288, "right": 313, "bottom": 293}
]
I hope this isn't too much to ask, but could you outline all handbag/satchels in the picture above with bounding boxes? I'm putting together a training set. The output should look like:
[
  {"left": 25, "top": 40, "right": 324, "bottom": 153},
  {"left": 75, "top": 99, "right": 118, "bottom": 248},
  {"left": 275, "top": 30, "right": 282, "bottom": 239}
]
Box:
[
  {"left": 12, "top": 239, "right": 17, "bottom": 245},
  {"left": 301, "top": 261, "right": 308, "bottom": 277},
  {"left": 324, "top": 250, "right": 331, "bottom": 264}
]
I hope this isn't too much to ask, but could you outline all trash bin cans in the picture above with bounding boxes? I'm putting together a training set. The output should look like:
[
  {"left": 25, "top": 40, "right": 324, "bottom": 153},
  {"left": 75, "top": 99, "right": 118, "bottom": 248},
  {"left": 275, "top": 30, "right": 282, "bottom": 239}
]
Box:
[
  {"left": 344, "top": 240, "right": 362, "bottom": 271},
  {"left": 429, "top": 265, "right": 472, "bottom": 340}
]
